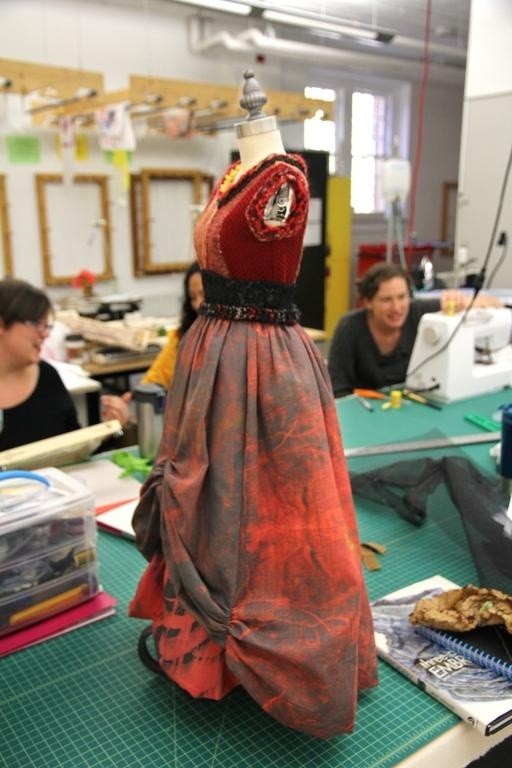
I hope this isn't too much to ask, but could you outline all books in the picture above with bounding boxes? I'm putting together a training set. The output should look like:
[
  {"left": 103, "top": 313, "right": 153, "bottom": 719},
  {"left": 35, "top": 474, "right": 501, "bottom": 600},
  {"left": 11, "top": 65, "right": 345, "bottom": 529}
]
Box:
[
  {"left": 413, "top": 620, "right": 512, "bottom": 679},
  {"left": 62, "top": 453, "right": 146, "bottom": 540},
  {"left": 366, "top": 573, "right": 512, "bottom": 738}
]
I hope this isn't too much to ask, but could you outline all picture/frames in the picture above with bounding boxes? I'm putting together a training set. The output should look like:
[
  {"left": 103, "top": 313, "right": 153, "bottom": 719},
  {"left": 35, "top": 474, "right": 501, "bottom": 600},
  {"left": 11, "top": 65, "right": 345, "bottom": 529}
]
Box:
[{"left": 0, "top": 169, "right": 214, "bottom": 286}]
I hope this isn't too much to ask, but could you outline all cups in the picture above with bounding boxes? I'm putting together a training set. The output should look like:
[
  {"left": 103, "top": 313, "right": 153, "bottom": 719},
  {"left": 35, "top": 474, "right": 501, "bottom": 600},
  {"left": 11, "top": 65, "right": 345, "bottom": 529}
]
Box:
[{"left": 65, "top": 334, "right": 85, "bottom": 364}]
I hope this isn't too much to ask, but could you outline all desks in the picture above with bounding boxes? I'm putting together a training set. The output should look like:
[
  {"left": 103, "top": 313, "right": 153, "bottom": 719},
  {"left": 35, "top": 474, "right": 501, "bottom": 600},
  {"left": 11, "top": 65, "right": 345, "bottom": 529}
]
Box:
[
  {"left": 0, "top": 383, "right": 512, "bottom": 767},
  {"left": 86, "top": 324, "right": 327, "bottom": 455}
]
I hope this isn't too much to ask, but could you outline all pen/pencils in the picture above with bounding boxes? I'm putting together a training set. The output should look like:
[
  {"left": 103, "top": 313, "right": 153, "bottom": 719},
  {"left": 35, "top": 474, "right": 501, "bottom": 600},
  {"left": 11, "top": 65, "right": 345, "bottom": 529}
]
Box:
[
  {"left": 353, "top": 393, "right": 375, "bottom": 412},
  {"left": 101, "top": 392, "right": 133, "bottom": 417}
]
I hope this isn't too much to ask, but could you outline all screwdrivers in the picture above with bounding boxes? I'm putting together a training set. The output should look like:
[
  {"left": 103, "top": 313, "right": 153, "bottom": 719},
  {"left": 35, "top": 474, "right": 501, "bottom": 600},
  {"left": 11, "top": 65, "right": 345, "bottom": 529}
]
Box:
[{"left": 351, "top": 388, "right": 441, "bottom": 411}]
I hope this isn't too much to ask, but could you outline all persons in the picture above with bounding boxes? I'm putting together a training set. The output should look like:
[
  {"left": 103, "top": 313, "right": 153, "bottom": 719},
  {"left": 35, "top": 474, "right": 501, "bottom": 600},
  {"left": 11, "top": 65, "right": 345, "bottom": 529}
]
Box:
[
  {"left": 127, "top": 104, "right": 381, "bottom": 736},
  {"left": 137, "top": 262, "right": 206, "bottom": 389},
  {"left": 1, "top": 277, "right": 136, "bottom": 453},
  {"left": 327, "top": 261, "right": 498, "bottom": 401}
]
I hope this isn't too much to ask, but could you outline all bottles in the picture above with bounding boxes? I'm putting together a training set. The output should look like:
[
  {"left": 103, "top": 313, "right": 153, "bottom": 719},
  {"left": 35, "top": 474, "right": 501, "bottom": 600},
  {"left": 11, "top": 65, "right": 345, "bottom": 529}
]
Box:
[{"left": 136, "top": 385, "right": 168, "bottom": 459}]
